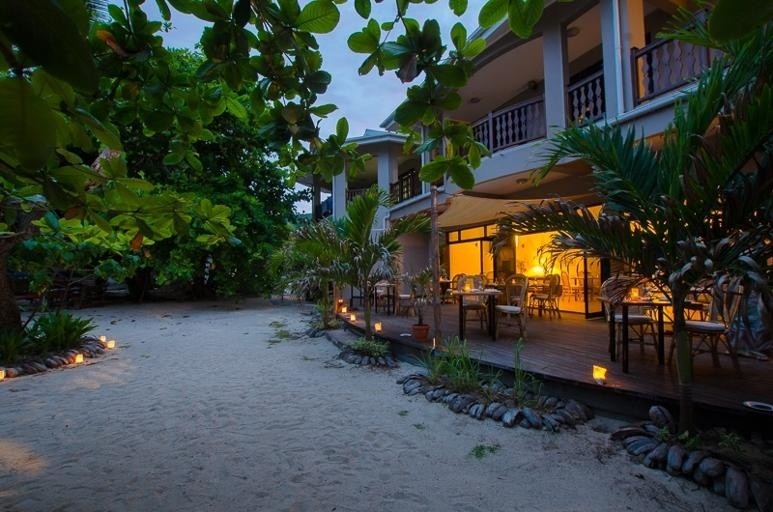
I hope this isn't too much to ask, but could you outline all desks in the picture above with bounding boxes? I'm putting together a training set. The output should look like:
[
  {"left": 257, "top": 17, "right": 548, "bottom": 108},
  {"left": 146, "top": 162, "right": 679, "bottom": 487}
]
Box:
[{"left": 350, "top": 283, "right": 368, "bottom": 307}]
